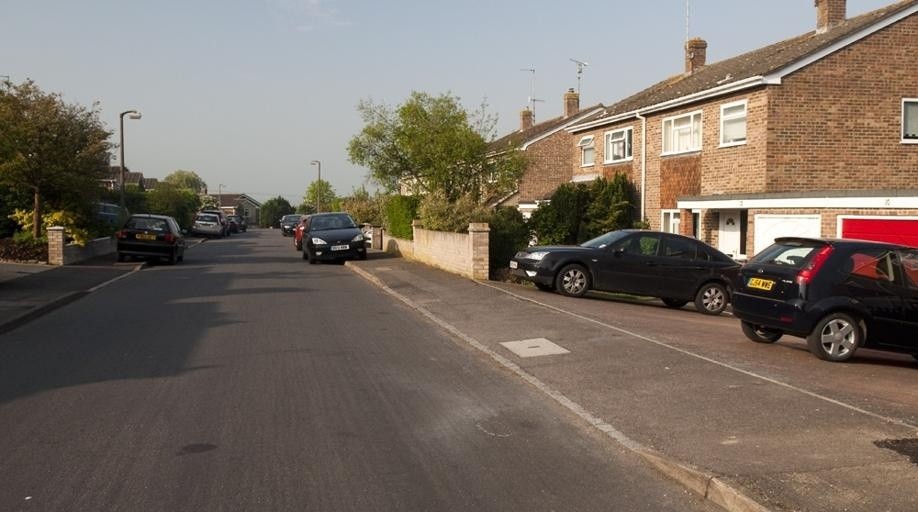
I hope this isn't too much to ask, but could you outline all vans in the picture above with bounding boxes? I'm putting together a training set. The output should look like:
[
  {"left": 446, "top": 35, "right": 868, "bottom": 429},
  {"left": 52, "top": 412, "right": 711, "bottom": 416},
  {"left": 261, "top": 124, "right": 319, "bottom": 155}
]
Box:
[{"left": 95, "top": 202, "right": 129, "bottom": 223}]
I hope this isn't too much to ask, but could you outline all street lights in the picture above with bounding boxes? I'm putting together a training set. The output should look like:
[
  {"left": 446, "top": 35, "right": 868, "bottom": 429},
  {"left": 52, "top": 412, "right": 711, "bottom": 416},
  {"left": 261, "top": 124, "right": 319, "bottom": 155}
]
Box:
[
  {"left": 310, "top": 160, "right": 321, "bottom": 214},
  {"left": 118, "top": 110, "right": 142, "bottom": 221},
  {"left": 217, "top": 183, "right": 227, "bottom": 211}
]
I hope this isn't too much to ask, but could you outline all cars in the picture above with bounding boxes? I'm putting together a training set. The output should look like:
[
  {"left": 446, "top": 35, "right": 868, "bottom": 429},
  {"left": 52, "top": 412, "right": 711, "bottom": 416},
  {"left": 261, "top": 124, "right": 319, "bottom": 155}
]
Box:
[
  {"left": 278, "top": 214, "right": 374, "bottom": 264},
  {"left": 731, "top": 236, "right": 917, "bottom": 362},
  {"left": 510, "top": 229, "right": 742, "bottom": 315},
  {"left": 115, "top": 214, "right": 188, "bottom": 264},
  {"left": 190, "top": 209, "right": 248, "bottom": 239}
]
